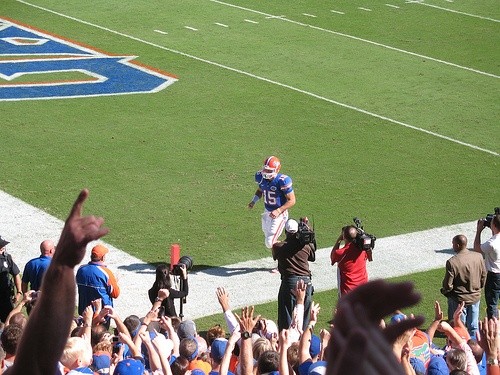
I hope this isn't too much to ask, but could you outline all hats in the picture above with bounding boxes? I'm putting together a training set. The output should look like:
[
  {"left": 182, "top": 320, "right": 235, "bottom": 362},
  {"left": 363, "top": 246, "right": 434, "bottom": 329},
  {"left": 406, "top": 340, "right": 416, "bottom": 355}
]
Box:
[
  {"left": 391, "top": 314, "right": 405, "bottom": 324},
  {"left": 445, "top": 326, "right": 470, "bottom": 344},
  {"left": 113, "top": 359, "right": 144, "bottom": 375},
  {"left": 410, "top": 358, "right": 426, "bottom": 375},
  {"left": 190, "top": 369, "right": 205, "bottom": 375},
  {"left": 192, "top": 360, "right": 212, "bottom": 375},
  {"left": 91, "top": 245, "right": 109, "bottom": 258},
  {"left": 286, "top": 218, "right": 299, "bottom": 233},
  {"left": 427, "top": 356, "right": 449, "bottom": 375},
  {"left": 310, "top": 335, "right": 321, "bottom": 355}
]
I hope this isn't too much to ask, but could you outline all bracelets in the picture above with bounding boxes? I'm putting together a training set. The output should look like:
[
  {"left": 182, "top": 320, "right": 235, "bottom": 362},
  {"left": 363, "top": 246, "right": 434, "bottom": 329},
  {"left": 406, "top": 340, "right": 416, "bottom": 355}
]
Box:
[
  {"left": 307, "top": 324, "right": 313, "bottom": 329},
  {"left": 276, "top": 208, "right": 281, "bottom": 214},
  {"left": 252, "top": 195, "right": 260, "bottom": 204}
]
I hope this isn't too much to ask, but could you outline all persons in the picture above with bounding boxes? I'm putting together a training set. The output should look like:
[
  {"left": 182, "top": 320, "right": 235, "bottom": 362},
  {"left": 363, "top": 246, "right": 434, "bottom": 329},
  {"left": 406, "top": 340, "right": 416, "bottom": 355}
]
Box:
[
  {"left": 148, "top": 263, "right": 188, "bottom": 318},
  {"left": 248, "top": 156, "right": 296, "bottom": 272},
  {"left": 21, "top": 239, "right": 55, "bottom": 316},
  {"left": 440, "top": 234, "right": 487, "bottom": 339},
  {"left": 75, "top": 245, "right": 120, "bottom": 331},
  {"left": 330, "top": 224, "right": 368, "bottom": 301},
  {"left": 0, "top": 189, "right": 500, "bottom": 375},
  {"left": 271, "top": 217, "right": 317, "bottom": 335}
]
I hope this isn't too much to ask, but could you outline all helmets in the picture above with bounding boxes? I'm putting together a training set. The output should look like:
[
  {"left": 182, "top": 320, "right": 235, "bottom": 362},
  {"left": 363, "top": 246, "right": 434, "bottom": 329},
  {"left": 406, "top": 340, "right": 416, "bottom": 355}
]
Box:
[{"left": 264, "top": 155, "right": 281, "bottom": 173}]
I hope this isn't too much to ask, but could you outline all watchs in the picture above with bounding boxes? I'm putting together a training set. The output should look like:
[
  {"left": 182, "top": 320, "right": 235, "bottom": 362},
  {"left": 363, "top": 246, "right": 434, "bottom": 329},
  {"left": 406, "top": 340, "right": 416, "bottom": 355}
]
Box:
[
  {"left": 16, "top": 291, "right": 22, "bottom": 294},
  {"left": 241, "top": 331, "right": 252, "bottom": 339},
  {"left": 487, "top": 358, "right": 498, "bottom": 364},
  {"left": 155, "top": 297, "right": 163, "bottom": 302}
]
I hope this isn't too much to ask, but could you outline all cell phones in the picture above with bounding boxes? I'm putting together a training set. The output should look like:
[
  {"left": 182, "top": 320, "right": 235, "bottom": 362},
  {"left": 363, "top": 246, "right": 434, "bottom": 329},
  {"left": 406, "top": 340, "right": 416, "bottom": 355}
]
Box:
[{"left": 158, "top": 306, "right": 165, "bottom": 319}]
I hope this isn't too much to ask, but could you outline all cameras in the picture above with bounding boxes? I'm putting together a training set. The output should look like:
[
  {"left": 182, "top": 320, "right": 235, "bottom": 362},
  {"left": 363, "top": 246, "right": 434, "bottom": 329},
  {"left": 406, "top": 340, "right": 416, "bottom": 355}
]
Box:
[
  {"left": 484, "top": 214, "right": 494, "bottom": 228},
  {"left": 32, "top": 292, "right": 39, "bottom": 299}
]
